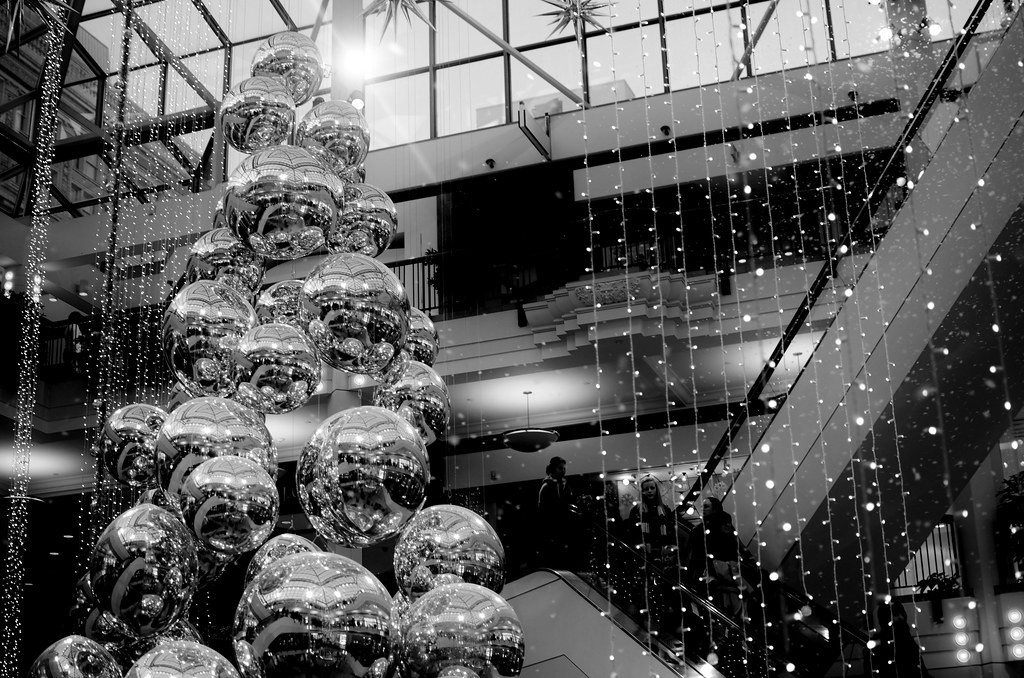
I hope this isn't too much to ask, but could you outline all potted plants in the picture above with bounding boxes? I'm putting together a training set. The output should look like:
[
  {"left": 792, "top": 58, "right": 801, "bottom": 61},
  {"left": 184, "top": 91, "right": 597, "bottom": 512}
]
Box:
[{"left": 902, "top": 574, "right": 1024, "bottom": 669}]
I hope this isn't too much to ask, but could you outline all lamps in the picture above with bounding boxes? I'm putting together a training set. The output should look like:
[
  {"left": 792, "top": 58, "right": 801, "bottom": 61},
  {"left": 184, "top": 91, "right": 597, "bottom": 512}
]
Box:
[{"left": 504, "top": 390, "right": 559, "bottom": 453}]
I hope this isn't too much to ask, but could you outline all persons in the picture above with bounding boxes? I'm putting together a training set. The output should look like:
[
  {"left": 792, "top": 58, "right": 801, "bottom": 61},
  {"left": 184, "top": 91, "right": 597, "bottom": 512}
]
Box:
[
  {"left": 684, "top": 497, "right": 750, "bottom": 666},
  {"left": 839, "top": 573, "right": 929, "bottom": 678},
  {"left": 537, "top": 457, "right": 699, "bottom": 662}
]
